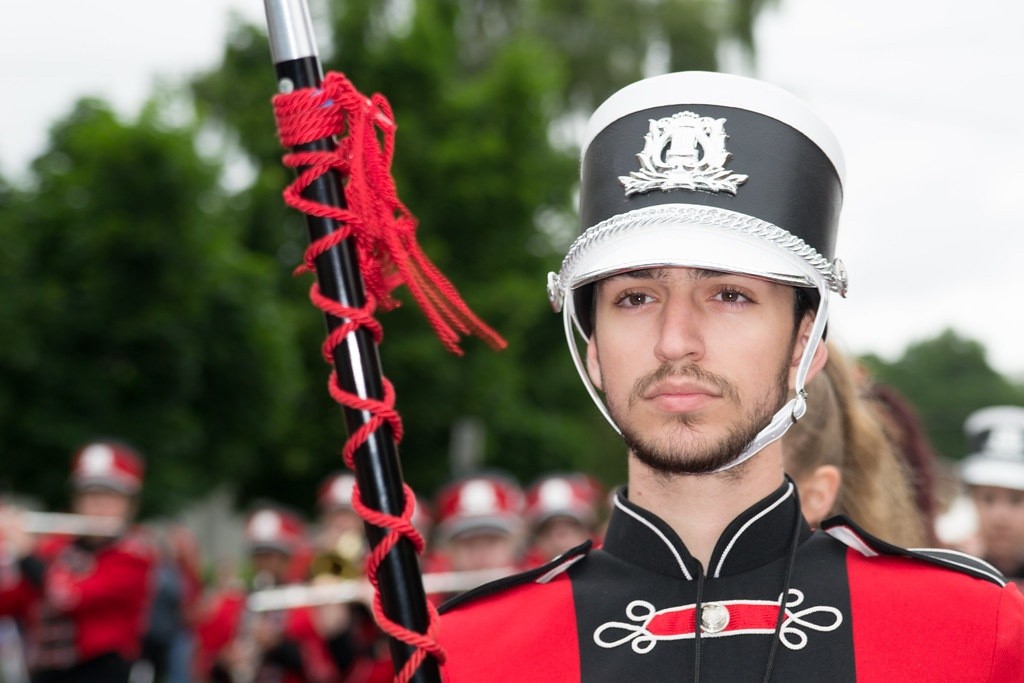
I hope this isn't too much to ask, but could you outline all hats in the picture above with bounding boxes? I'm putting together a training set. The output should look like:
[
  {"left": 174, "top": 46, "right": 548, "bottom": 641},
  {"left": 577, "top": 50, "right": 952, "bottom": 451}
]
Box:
[
  {"left": 962, "top": 405, "right": 1024, "bottom": 489},
  {"left": 545, "top": 69, "right": 848, "bottom": 344},
  {"left": 74, "top": 441, "right": 611, "bottom": 553}
]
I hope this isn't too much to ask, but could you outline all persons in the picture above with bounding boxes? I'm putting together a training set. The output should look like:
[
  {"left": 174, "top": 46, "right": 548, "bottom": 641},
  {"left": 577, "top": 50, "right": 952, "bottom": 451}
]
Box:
[
  {"left": 436, "top": 71, "right": 1024, "bottom": 683},
  {"left": 782, "top": 343, "right": 1024, "bottom": 595},
  {"left": 0, "top": 445, "right": 606, "bottom": 683}
]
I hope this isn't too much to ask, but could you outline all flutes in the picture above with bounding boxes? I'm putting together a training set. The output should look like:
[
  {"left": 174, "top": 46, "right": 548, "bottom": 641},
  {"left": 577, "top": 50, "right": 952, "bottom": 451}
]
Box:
[
  {"left": 19, "top": 510, "right": 126, "bottom": 537},
  {"left": 246, "top": 564, "right": 520, "bottom": 613}
]
